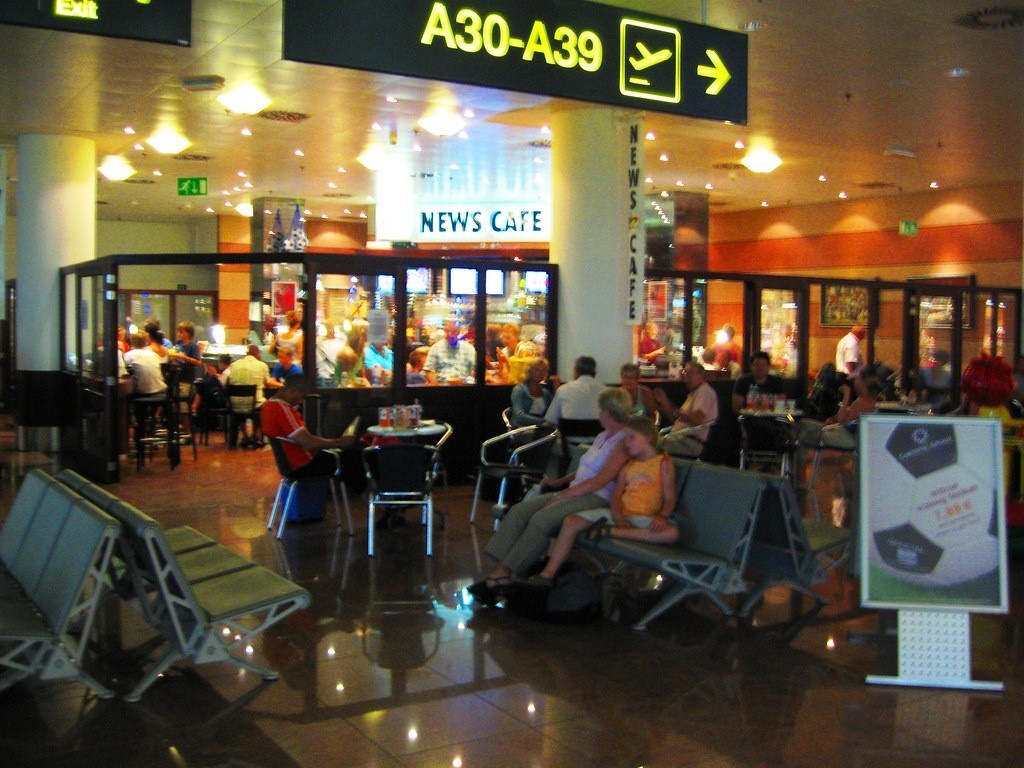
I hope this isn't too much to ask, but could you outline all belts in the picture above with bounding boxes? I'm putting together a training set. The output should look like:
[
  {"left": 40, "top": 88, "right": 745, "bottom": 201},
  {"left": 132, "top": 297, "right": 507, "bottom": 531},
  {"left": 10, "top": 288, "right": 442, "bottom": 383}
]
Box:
[{"left": 686, "top": 435, "right": 706, "bottom": 445}]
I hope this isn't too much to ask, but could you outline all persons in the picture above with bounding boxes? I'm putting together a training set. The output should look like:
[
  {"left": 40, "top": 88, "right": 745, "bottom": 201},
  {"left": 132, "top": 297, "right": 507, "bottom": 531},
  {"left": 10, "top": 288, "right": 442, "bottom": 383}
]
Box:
[
  {"left": 261, "top": 374, "right": 405, "bottom": 528},
  {"left": 512, "top": 326, "right": 1024, "bottom": 459},
  {"left": 97, "top": 317, "right": 535, "bottom": 456},
  {"left": 527, "top": 418, "right": 680, "bottom": 586},
  {"left": 466, "top": 386, "right": 633, "bottom": 602}
]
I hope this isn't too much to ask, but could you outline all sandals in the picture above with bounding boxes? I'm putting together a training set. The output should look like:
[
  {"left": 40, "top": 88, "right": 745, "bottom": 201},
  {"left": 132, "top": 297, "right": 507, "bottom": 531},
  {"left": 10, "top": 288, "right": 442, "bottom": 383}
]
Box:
[
  {"left": 515, "top": 574, "right": 551, "bottom": 590},
  {"left": 585, "top": 517, "right": 613, "bottom": 541}
]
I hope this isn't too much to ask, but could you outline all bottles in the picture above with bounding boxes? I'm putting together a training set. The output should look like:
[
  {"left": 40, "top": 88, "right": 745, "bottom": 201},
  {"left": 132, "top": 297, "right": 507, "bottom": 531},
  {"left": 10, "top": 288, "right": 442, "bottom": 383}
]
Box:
[{"left": 745, "top": 384, "right": 796, "bottom": 412}]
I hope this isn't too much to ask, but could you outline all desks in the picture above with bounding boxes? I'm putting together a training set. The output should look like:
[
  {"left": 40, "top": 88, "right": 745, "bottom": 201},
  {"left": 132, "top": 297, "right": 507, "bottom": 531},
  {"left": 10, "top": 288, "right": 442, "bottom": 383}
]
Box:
[
  {"left": 367, "top": 425, "right": 447, "bottom": 529},
  {"left": 876, "top": 402, "right": 932, "bottom": 412}
]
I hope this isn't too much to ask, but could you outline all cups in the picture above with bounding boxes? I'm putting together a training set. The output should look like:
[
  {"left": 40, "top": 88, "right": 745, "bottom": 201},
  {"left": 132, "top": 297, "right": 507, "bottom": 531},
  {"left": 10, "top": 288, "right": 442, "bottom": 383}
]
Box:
[
  {"left": 639, "top": 366, "right": 658, "bottom": 376},
  {"left": 378, "top": 405, "right": 421, "bottom": 433}
]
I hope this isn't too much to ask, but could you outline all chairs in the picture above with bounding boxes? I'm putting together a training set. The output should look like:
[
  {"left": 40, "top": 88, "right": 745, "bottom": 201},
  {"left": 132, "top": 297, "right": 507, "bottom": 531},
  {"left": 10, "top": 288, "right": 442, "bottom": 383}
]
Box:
[
  {"left": 468, "top": 407, "right": 604, "bottom": 533},
  {"left": 268, "top": 421, "right": 453, "bottom": 556},
  {"left": 652, "top": 409, "right": 859, "bottom": 495},
  {"left": 193, "top": 380, "right": 280, "bottom": 450}
]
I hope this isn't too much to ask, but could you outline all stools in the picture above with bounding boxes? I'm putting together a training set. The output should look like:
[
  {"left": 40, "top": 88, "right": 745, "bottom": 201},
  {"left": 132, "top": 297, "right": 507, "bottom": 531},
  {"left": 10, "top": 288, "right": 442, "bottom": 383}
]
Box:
[{"left": 130, "top": 363, "right": 198, "bottom": 470}]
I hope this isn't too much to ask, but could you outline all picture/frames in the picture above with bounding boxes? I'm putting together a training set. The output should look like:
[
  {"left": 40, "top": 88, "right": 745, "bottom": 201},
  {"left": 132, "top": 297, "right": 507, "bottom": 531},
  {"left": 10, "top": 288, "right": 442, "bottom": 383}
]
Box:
[
  {"left": 820, "top": 276, "right": 879, "bottom": 328},
  {"left": 906, "top": 274, "right": 976, "bottom": 329}
]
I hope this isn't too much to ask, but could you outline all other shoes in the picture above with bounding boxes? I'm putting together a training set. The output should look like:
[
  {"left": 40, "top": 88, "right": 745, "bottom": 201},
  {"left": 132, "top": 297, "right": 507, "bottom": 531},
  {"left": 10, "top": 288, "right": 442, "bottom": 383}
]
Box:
[
  {"left": 467, "top": 574, "right": 516, "bottom": 604},
  {"left": 239, "top": 437, "right": 253, "bottom": 448}
]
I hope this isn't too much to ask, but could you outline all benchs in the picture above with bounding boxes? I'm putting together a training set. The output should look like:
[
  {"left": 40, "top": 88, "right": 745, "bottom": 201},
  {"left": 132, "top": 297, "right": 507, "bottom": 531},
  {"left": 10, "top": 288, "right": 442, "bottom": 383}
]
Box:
[
  {"left": 488, "top": 443, "right": 852, "bottom": 630},
  {"left": 0, "top": 468, "right": 313, "bottom": 701}
]
